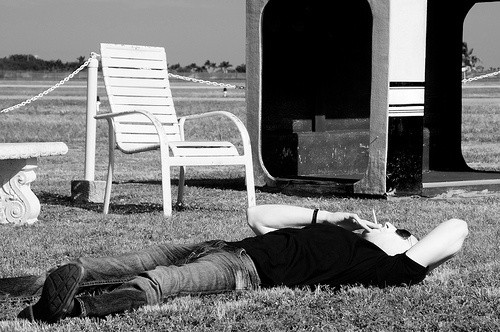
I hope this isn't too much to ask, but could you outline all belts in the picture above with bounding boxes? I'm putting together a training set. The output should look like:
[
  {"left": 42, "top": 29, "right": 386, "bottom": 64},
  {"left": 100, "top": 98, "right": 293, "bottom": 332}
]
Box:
[{"left": 203, "top": 239, "right": 259, "bottom": 290}]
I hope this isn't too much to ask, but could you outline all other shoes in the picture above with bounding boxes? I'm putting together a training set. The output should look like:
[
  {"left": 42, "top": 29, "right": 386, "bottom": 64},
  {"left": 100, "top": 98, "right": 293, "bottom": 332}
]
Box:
[{"left": 16, "top": 264, "right": 82, "bottom": 323}]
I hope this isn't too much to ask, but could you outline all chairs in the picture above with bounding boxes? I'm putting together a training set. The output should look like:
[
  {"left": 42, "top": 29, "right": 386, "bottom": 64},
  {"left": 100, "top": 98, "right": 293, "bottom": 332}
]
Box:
[{"left": 93, "top": 43, "right": 256, "bottom": 217}]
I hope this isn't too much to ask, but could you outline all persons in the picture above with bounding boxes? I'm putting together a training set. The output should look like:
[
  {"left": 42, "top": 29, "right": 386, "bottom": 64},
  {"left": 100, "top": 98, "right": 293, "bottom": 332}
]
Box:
[{"left": 17, "top": 207, "right": 468, "bottom": 323}]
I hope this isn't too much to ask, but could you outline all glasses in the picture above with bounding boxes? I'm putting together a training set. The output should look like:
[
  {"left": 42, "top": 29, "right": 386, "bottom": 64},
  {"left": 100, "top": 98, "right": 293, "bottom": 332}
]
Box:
[{"left": 395, "top": 229, "right": 413, "bottom": 248}]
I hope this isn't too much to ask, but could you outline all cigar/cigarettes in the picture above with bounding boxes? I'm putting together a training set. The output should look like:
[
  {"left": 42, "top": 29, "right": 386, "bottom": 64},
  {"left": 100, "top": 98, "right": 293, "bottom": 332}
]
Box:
[{"left": 372, "top": 208, "right": 378, "bottom": 224}]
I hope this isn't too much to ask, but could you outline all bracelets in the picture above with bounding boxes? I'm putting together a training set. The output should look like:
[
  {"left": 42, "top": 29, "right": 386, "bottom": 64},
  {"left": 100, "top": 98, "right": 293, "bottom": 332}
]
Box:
[{"left": 311, "top": 209, "right": 319, "bottom": 222}]
